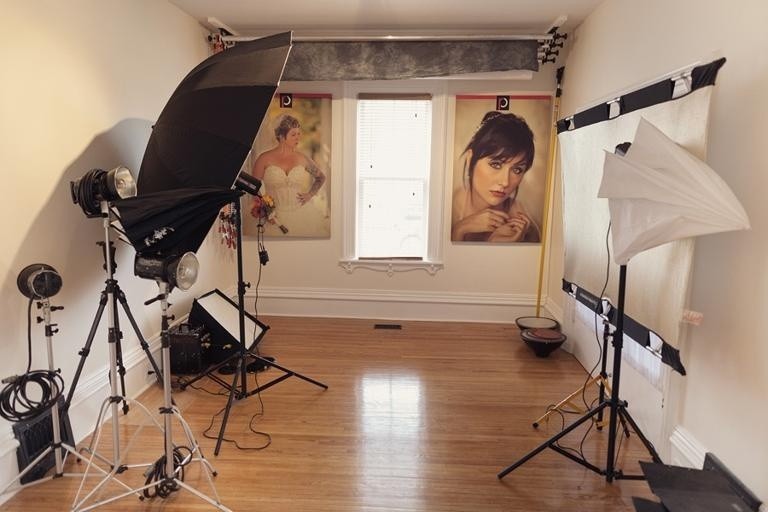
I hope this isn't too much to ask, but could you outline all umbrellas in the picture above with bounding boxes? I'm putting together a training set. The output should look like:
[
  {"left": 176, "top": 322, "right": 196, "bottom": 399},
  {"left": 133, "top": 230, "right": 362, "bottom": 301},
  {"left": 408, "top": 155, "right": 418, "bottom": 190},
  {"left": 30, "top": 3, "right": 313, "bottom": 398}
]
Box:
[
  {"left": 596, "top": 114, "right": 751, "bottom": 401},
  {"left": 107, "top": 184, "right": 246, "bottom": 258},
  {"left": 134, "top": 31, "right": 294, "bottom": 350}
]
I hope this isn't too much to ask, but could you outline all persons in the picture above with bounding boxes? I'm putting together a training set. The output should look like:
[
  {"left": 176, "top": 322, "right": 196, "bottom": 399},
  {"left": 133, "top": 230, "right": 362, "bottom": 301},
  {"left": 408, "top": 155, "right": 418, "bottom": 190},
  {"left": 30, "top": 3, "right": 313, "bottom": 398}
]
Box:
[
  {"left": 451, "top": 107, "right": 539, "bottom": 241},
  {"left": 253, "top": 115, "right": 326, "bottom": 237}
]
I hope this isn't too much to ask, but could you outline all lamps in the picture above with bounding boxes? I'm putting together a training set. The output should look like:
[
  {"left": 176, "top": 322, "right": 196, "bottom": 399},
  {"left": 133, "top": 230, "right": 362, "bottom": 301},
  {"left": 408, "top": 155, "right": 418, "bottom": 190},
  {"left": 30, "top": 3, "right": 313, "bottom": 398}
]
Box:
[
  {"left": 17, "top": 263, "right": 64, "bottom": 299},
  {"left": 134, "top": 251, "right": 199, "bottom": 294},
  {"left": 69, "top": 166, "right": 138, "bottom": 216},
  {"left": 235, "top": 173, "right": 263, "bottom": 193},
  {"left": 187, "top": 287, "right": 269, "bottom": 366}
]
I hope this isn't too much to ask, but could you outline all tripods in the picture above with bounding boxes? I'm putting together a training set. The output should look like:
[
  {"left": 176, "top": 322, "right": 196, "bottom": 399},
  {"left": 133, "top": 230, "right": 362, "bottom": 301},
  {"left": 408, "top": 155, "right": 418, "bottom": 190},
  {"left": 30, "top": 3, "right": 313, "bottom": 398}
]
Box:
[
  {"left": 0, "top": 197, "right": 327, "bottom": 512},
  {"left": 533, "top": 322, "right": 630, "bottom": 438},
  {"left": 497, "top": 265, "right": 664, "bottom": 482}
]
